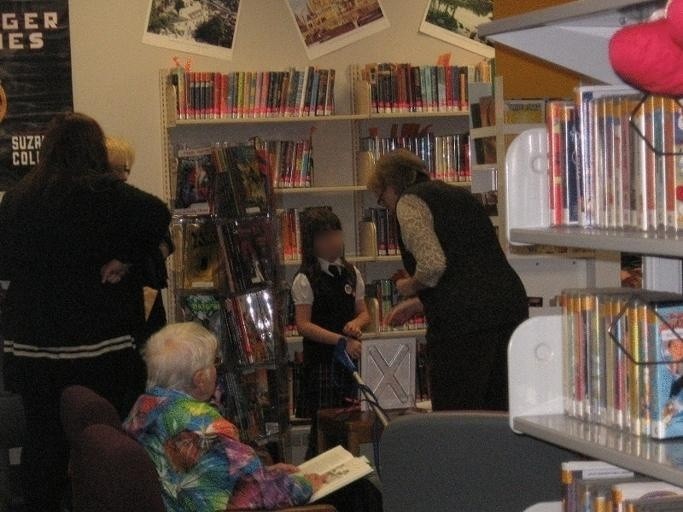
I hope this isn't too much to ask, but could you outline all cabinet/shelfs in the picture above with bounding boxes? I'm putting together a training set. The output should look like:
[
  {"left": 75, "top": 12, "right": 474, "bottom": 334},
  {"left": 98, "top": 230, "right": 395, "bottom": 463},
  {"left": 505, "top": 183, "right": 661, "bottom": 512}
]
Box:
[
  {"left": 152, "top": 54, "right": 507, "bottom": 453},
  {"left": 487, "top": 61, "right": 683, "bottom": 511}
]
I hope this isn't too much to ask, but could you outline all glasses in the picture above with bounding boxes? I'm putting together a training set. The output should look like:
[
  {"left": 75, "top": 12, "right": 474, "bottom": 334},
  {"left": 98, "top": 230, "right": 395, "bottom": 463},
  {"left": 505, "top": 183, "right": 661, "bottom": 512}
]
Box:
[{"left": 376, "top": 192, "right": 390, "bottom": 209}]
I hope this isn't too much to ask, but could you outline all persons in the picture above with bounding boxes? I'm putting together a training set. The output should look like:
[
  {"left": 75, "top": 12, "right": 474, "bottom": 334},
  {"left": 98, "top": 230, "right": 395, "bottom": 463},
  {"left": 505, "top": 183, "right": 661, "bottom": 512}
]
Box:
[
  {"left": 123, "top": 322, "right": 382, "bottom": 512},
  {"left": 366, "top": 149, "right": 529, "bottom": 411},
  {"left": 105, "top": 136, "right": 176, "bottom": 346},
  {"left": 0, "top": 111, "right": 172, "bottom": 512}
]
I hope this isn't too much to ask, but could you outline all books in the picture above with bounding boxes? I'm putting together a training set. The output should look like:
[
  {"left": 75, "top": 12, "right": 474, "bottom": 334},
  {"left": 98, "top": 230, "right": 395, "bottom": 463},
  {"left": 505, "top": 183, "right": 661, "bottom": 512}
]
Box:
[
  {"left": 546, "top": 85, "right": 683, "bottom": 234},
  {"left": 416, "top": 340, "right": 432, "bottom": 402},
  {"left": 562, "top": 461, "right": 683, "bottom": 512},
  {"left": 170, "top": 136, "right": 312, "bottom": 197},
  {"left": 170, "top": 208, "right": 301, "bottom": 288},
  {"left": 359, "top": 63, "right": 469, "bottom": 332},
  {"left": 359, "top": 124, "right": 471, "bottom": 185},
  {"left": 180, "top": 289, "right": 302, "bottom": 338},
  {"left": 214, "top": 360, "right": 314, "bottom": 467},
  {"left": 170, "top": 67, "right": 335, "bottom": 118},
  {"left": 561, "top": 289, "right": 683, "bottom": 441}
]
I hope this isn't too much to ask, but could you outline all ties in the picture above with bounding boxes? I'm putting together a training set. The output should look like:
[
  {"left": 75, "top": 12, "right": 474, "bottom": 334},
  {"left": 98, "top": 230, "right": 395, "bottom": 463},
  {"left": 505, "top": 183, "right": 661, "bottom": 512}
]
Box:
[{"left": 329, "top": 264, "right": 341, "bottom": 277}]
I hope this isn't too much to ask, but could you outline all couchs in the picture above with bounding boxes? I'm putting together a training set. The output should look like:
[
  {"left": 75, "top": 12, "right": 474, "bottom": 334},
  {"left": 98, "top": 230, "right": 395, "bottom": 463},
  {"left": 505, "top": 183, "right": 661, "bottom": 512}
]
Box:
[{"left": 58, "top": 385, "right": 346, "bottom": 510}]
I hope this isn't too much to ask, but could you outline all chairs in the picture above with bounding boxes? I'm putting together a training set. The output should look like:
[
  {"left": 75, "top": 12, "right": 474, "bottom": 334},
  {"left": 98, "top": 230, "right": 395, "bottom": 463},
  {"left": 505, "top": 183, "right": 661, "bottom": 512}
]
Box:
[{"left": 378, "top": 410, "right": 568, "bottom": 511}]
